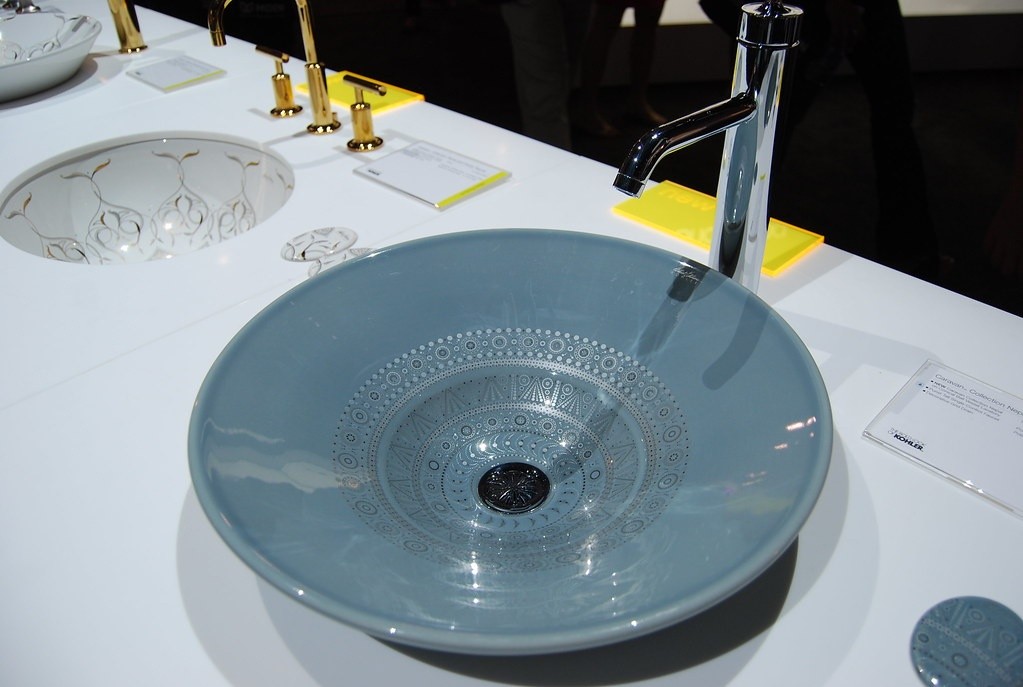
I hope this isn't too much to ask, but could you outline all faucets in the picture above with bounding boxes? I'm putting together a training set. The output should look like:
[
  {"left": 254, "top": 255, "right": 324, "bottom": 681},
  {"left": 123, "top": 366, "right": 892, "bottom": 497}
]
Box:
[
  {"left": 613, "top": 1, "right": 804, "bottom": 296},
  {"left": 209, "top": 0, "right": 341, "bottom": 134}
]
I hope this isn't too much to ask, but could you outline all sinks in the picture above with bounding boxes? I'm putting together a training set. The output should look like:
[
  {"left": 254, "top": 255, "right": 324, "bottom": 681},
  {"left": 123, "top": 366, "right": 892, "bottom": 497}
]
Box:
[
  {"left": 188, "top": 228, "right": 835, "bottom": 655},
  {"left": 0, "top": 12, "right": 102, "bottom": 104},
  {"left": 0, "top": 129, "right": 295, "bottom": 267}
]
